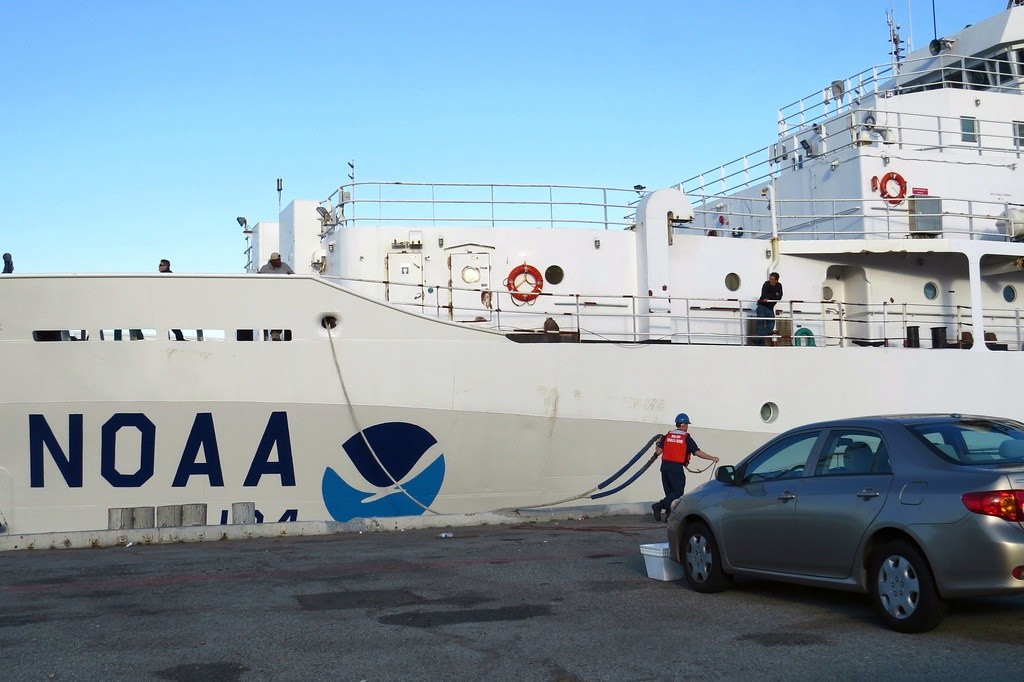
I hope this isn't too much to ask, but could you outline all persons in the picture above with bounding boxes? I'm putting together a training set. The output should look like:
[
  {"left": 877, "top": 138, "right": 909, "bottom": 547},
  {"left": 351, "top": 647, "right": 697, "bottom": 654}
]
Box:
[
  {"left": 752, "top": 271, "right": 783, "bottom": 346},
  {"left": 651, "top": 414, "right": 719, "bottom": 523},
  {"left": 258, "top": 253, "right": 295, "bottom": 341},
  {"left": 157, "top": 259, "right": 187, "bottom": 341}
]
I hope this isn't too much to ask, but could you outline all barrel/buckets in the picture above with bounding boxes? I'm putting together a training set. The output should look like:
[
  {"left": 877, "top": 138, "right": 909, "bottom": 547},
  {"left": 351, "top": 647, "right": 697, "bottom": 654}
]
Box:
[
  {"left": 182, "top": 504, "right": 207, "bottom": 526},
  {"left": 156, "top": 505, "right": 183, "bottom": 528},
  {"left": 930, "top": 327, "right": 948, "bottom": 348},
  {"left": 231, "top": 502, "right": 255, "bottom": 523},
  {"left": 134, "top": 507, "right": 156, "bottom": 528},
  {"left": 902, "top": 326, "right": 920, "bottom": 348},
  {"left": 108, "top": 508, "right": 134, "bottom": 530}
]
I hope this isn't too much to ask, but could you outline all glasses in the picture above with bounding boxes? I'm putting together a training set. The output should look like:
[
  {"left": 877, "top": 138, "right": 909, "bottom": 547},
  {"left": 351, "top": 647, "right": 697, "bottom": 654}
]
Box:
[{"left": 159, "top": 264, "right": 166, "bottom": 267}]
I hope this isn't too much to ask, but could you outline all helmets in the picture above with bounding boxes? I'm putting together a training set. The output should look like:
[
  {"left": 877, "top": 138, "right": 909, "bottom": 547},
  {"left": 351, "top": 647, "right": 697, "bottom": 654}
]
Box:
[{"left": 674, "top": 413, "right": 691, "bottom": 424}]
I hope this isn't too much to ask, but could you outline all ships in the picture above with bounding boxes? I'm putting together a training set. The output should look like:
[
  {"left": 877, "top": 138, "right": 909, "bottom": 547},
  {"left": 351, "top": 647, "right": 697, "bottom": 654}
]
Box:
[{"left": 0, "top": 0, "right": 1024, "bottom": 552}]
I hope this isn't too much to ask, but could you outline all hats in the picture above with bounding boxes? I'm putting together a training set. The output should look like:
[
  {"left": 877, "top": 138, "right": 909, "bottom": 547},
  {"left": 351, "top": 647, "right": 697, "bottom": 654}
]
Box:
[{"left": 268, "top": 252, "right": 281, "bottom": 262}]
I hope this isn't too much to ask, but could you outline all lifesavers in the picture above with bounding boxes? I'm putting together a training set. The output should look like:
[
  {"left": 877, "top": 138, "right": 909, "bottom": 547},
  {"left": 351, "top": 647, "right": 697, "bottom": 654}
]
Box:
[
  {"left": 506, "top": 265, "right": 542, "bottom": 301},
  {"left": 882, "top": 171, "right": 906, "bottom": 204}
]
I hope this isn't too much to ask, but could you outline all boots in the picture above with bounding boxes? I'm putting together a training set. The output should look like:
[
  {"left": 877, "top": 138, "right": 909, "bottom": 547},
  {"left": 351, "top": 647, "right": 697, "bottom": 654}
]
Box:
[
  {"left": 651, "top": 502, "right": 662, "bottom": 521},
  {"left": 665, "top": 508, "right": 671, "bottom": 523}
]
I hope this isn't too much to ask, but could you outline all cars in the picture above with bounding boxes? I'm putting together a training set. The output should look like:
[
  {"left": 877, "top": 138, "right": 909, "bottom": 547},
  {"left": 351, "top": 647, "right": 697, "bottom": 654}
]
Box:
[{"left": 666, "top": 412, "right": 1024, "bottom": 636}]
